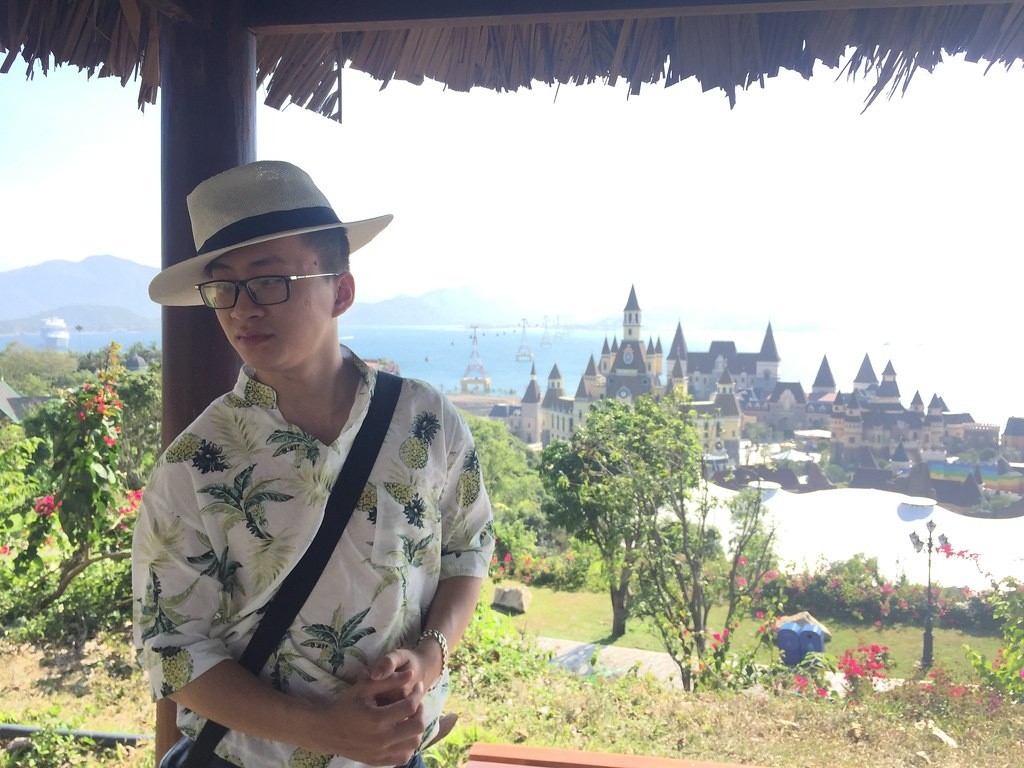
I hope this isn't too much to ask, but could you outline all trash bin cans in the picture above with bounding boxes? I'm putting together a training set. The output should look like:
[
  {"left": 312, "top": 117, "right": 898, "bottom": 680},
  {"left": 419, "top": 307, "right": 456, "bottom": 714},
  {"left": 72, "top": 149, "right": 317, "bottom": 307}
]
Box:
[
  {"left": 778, "top": 622, "right": 800, "bottom": 666},
  {"left": 799, "top": 624, "right": 825, "bottom": 672}
]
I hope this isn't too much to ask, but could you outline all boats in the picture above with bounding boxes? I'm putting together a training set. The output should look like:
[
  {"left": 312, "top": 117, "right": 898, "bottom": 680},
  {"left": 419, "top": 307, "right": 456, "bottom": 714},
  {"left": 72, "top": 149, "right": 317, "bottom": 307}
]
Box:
[{"left": 40, "top": 317, "right": 70, "bottom": 348}]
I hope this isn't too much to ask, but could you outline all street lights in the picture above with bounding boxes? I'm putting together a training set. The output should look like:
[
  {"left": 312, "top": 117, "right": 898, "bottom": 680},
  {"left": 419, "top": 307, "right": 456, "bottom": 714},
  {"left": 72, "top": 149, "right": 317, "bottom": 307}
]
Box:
[{"left": 920, "top": 520, "right": 936, "bottom": 669}]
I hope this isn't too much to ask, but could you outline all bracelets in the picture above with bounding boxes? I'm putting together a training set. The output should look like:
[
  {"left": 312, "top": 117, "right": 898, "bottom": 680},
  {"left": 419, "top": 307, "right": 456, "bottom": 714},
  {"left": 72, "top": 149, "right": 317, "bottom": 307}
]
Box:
[{"left": 417, "top": 629, "right": 448, "bottom": 691}]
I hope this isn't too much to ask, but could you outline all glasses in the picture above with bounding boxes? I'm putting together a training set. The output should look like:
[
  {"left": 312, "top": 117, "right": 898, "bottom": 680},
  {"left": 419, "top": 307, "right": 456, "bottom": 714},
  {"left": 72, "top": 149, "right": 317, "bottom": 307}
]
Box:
[{"left": 195, "top": 273, "right": 339, "bottom": 310}]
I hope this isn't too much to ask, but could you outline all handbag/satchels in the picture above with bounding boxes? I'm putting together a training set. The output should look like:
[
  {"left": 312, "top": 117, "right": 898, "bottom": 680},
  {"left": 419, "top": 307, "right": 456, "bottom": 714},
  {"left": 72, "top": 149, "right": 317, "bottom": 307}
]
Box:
[{"left": 157, "top": 736, "right": 195, "bottom": 768}]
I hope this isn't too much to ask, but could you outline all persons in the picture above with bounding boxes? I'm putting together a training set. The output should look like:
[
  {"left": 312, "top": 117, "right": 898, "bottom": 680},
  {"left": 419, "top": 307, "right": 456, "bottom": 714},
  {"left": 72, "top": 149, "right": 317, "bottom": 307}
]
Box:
[{"left": 130, "top": 162, "right": 496, "bottom": 768}]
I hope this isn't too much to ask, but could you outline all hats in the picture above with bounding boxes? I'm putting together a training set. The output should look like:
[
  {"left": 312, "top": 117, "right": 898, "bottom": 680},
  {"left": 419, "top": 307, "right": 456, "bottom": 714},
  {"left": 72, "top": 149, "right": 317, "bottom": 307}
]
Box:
[{"left": 148, "top": 161, "right": 394, "bottom": 307}]
